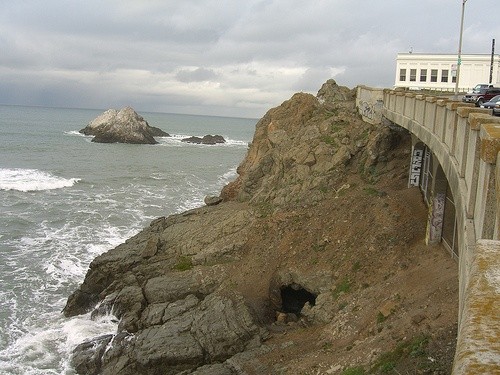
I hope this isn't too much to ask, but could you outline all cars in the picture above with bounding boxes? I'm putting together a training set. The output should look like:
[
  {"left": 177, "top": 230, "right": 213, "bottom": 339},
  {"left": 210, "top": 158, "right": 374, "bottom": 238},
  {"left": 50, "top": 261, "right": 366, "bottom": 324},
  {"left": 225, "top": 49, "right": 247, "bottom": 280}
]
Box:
[{"left": 481, "top": 95, "right": 500, "bottom": 116}]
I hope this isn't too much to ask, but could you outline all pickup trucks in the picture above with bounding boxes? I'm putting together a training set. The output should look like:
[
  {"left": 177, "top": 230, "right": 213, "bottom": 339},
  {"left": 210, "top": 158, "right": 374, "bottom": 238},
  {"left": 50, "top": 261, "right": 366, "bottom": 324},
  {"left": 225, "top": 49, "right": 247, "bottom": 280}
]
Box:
[{"left": 462, "top": 84, "right": 500, "bottom": 100}]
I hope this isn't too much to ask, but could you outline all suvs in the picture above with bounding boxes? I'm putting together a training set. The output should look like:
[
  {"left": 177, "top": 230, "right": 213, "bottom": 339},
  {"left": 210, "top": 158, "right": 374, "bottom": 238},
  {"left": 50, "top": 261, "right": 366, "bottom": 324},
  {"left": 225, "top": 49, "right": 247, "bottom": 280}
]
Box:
[{"left": 465, "top": 89, "right": 500, "bottom": 107}]
